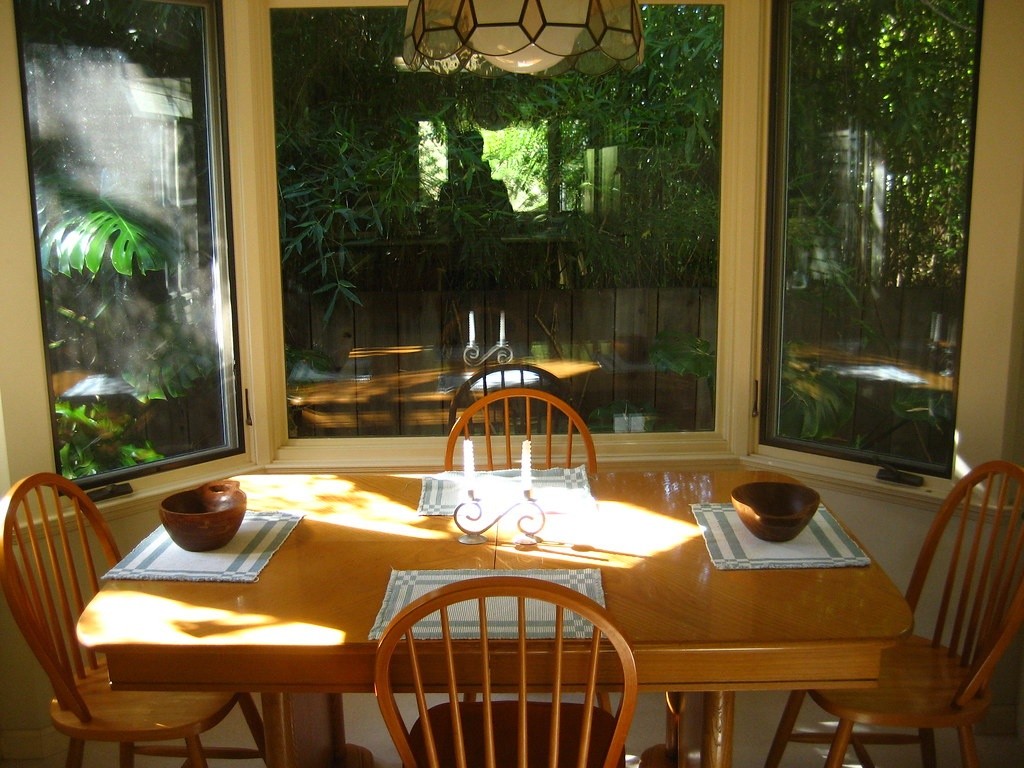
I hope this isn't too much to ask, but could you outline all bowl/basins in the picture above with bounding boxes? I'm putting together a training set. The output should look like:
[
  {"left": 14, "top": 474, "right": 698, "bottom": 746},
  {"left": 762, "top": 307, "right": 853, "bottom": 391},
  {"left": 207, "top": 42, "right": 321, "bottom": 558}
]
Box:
[
  {"left": 731, "top": 480, "right": 820, "bottom": 543},
  {"left": 157, "top": 477, "right": 248, "bottom": 551}
]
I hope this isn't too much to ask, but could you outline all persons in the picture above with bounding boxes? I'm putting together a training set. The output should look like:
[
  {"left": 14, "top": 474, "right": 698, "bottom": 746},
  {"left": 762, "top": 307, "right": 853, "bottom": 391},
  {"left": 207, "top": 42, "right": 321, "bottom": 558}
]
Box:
[{"left": 439, "top": 131, "right": 514, "bottom": 364}]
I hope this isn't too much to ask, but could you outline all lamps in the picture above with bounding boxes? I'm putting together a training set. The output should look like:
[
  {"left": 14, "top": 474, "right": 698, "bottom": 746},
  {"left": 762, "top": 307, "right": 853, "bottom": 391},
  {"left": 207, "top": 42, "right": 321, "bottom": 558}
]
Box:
[{"left": 402, "top": 0, "right": 645, "bottom": 78}]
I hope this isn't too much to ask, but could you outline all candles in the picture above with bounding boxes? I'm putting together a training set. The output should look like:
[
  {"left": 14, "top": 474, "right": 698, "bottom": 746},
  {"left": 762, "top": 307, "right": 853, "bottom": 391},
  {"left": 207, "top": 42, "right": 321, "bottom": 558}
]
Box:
[
  {"left": 469, "top": 311, "right": 475, "bottom": 340},
  {"left": 521, "top": 439, "right": 532, "bottom": 490},
  {"left": 463, "top": 438, "right": 476, "bottom": 490},
  {"left": 500, "top": 312, "right": 505, "bottom": 339}
]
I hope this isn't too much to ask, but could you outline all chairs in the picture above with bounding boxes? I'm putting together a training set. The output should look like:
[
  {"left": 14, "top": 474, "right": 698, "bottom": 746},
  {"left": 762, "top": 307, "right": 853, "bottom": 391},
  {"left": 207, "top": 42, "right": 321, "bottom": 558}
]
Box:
[
  {"left": 0, "top": 471, "right": 267, "bottom": 768},
  {"left": 373, "top": 576, "right": 638, "bottom": 768},
  {"left": 444, "top": 388, "right": 613, "bottom": 716},
  {"left": 764, "top": 460, "right": 1024, "bottom": 768}
]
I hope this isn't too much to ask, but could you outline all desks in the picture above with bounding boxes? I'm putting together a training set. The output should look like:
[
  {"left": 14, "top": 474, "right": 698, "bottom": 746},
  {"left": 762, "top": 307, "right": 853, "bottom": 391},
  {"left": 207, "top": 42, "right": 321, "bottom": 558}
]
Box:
[
  {"left": 287, "top": 356, "right": 602, "bottom": 434},
  {"left": 76, "top": 471, "right": 914, "bottom": 768}
]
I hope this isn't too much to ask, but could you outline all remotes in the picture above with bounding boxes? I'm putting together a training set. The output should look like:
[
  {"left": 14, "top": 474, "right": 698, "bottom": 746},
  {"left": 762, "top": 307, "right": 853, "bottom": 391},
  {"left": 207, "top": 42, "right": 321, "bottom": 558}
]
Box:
[{"left": 876, "top": 469, "right": 923, "bottom": 487}]
